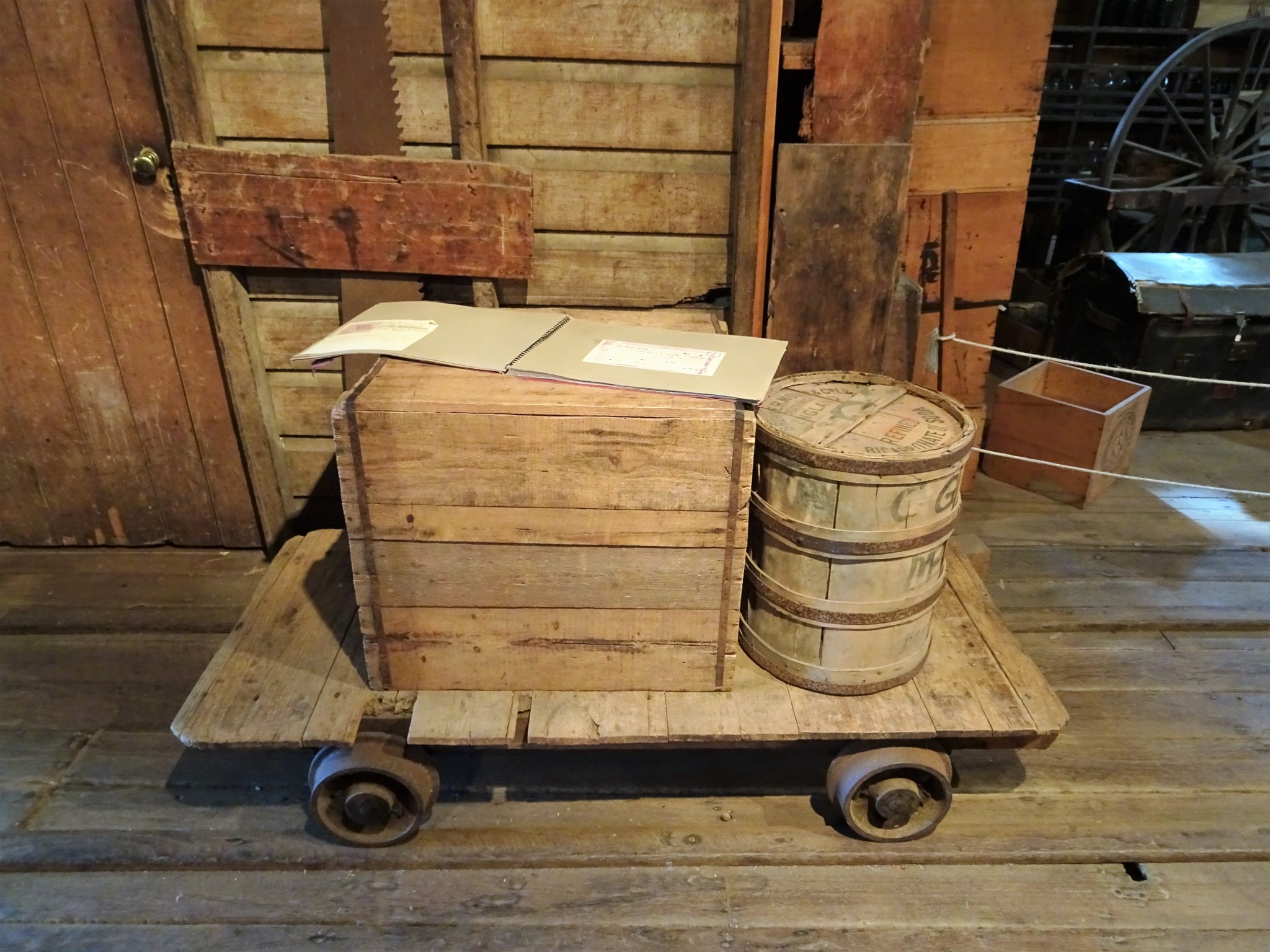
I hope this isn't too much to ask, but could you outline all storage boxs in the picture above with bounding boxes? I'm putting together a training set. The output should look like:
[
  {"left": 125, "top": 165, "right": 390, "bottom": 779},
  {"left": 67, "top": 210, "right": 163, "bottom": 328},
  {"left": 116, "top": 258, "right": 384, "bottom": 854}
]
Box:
[
  {"left": 976, "top": 360, "right": 1154, "bottom": 513},
  {"left": 323, "top": 300, "right": 762, "bottom": 701},
  {"left": 1047, "top": 251, "right": 1270, "bottom": 440}
]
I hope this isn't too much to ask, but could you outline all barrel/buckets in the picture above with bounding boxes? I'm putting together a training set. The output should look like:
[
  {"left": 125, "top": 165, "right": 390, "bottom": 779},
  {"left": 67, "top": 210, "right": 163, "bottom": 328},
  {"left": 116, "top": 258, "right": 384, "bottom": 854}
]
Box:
[{"left": 737, "top": 371, "right": 975, "bottom": 697}]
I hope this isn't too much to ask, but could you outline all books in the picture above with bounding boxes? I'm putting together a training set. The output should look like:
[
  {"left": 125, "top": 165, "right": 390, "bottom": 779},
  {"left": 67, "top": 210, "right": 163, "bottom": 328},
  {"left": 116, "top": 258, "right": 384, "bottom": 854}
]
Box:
[{"left": 288, "top": 301, "right": 789, "bottom": 405}]
